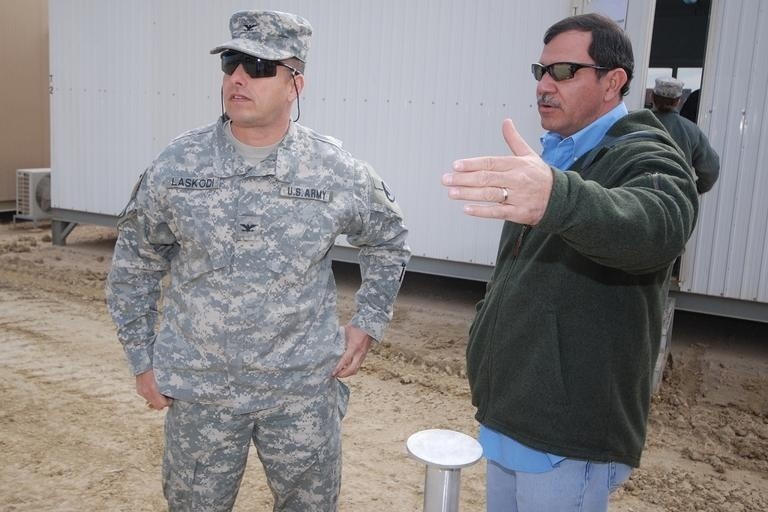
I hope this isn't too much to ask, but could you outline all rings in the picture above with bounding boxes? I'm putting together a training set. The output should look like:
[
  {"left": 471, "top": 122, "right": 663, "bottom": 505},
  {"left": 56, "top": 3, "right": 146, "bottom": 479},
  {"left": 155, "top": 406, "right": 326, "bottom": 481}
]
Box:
[
  {"left": 498, "top": 187, "right": 509, "bottom": 204},
  {"left": 146, "top": 399, "right": 154, "bottom": 409}
]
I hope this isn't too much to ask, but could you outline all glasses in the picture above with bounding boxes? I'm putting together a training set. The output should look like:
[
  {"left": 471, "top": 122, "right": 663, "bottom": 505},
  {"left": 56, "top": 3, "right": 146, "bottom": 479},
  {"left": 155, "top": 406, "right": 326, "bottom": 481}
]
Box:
[
  {"left": 220, "top": 49, "right": 302, "bottom": 78},
  {"left": 531, "top": 62, "right": 614, "bottom": 82}
]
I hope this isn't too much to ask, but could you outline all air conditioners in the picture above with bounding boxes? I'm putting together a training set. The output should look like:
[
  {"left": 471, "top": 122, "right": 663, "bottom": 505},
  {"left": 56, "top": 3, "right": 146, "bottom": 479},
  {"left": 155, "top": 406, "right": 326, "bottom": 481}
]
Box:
[{"left": 12, "top": 167, "right": 51, "bottom": 230}]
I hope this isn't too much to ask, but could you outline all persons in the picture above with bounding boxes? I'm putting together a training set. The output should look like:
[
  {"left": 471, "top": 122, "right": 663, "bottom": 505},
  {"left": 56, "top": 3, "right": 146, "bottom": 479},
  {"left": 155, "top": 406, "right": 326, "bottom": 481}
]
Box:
[
  {"left": 102, "top": 7, "right": 410, "bottom": 511},
  {"left": 645, "top": 75, "right": 721, "bottom": 317},
  {"left": 437, "top": 12, "right": 701, "bottom": 510}
]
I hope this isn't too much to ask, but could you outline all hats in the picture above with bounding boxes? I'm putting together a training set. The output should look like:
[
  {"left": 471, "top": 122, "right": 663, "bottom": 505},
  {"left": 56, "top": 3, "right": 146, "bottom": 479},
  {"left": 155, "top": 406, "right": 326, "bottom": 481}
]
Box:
[
  {"left": 653, "top": 77, "right": 685, "bottom": 98},
  {"left": 209, "top": 8, "right": 312, "bottom": 65}
]
